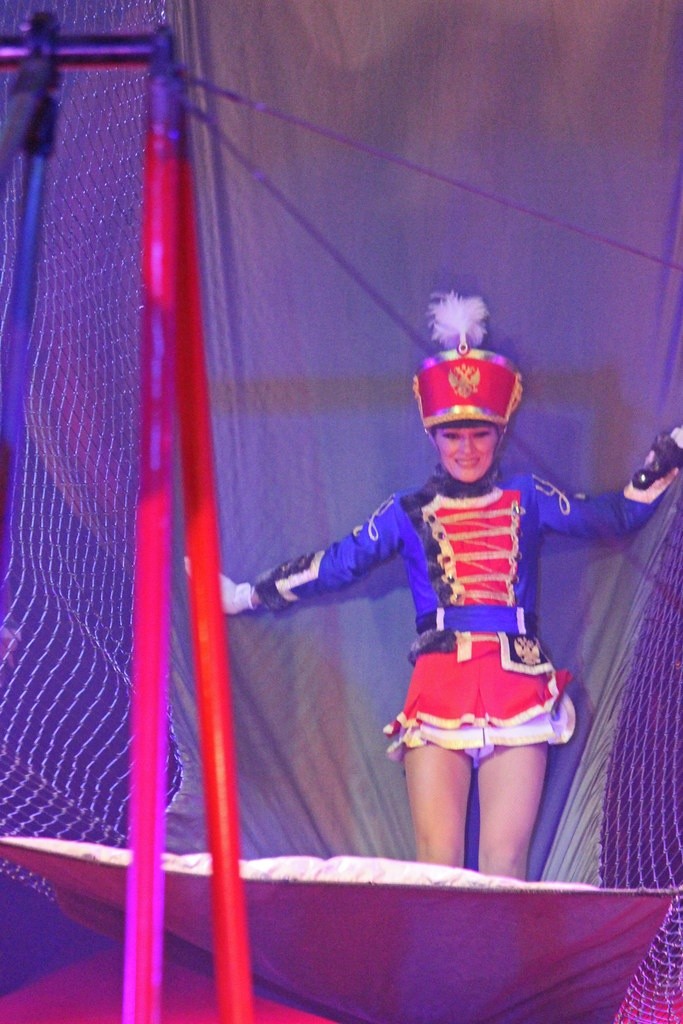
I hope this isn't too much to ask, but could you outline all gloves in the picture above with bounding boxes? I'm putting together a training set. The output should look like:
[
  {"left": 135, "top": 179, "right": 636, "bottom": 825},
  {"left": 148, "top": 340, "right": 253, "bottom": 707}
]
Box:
[{"left": 183, "top": 556, "right": 254, "bottom": 616}]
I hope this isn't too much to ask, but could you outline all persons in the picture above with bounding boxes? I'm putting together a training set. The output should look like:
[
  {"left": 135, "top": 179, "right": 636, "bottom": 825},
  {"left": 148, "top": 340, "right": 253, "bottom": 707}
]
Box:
[{"left": 182, "top": 290, "right": 683, "bottom": 882}]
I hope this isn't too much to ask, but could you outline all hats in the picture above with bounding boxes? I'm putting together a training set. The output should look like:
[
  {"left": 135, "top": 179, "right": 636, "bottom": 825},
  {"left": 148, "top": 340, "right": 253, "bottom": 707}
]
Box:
[{"left": 412, "top": 291, "right": 522, "bottom": 432}]
